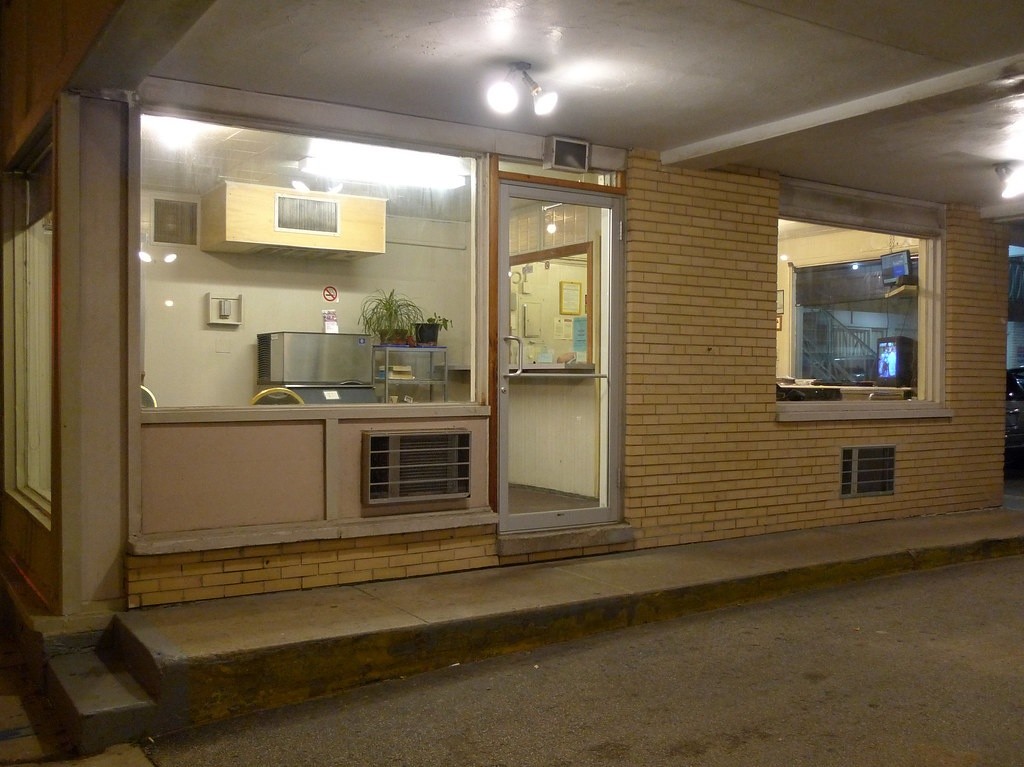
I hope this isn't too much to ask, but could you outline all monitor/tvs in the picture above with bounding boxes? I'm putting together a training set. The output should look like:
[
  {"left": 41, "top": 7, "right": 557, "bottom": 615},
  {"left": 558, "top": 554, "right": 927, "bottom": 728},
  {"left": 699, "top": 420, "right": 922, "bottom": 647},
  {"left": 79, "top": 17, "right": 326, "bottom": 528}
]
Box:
[
  {"left": 880, "top": 249, "right": 912, "bottom": 285},
  {"left": 876, "top": 335, "right": 919, "bottom": 388}
]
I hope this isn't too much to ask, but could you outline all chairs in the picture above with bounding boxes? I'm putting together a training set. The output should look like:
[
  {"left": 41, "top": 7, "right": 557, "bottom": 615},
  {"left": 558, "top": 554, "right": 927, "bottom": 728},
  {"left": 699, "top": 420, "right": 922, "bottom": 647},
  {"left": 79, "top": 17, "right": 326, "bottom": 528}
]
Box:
[{"left": 248, "top": 386, "right": 305, "bottom": 406}]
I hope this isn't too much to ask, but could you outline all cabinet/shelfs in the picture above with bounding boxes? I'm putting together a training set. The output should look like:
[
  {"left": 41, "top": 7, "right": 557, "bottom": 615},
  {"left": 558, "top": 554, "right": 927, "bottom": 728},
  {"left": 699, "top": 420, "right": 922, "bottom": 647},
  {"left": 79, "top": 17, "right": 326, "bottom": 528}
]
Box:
[{"left": 372, "top": 345, "right": 448, "bottom": 403}]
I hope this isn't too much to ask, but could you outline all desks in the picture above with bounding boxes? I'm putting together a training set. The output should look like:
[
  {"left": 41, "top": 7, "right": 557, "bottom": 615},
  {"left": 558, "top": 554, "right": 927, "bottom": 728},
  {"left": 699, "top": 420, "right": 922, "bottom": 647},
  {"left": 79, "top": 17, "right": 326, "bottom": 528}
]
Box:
[{"left": 777, "top": 383, "right": 913, "bottom": 399}]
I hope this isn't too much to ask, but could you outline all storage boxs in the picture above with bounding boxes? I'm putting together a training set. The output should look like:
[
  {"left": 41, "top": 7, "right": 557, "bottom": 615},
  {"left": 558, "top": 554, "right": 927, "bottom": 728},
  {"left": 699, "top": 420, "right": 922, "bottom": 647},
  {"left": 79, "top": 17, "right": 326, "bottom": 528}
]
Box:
[{"left": 380, "top": 366, "right": 412, "bottom": 380}]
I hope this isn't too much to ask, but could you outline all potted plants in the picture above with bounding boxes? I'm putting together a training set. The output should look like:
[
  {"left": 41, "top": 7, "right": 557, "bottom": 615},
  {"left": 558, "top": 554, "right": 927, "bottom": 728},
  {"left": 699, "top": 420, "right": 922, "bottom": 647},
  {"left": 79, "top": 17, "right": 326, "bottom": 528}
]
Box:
[{"left": 357, "top": 289, "right": 453, "bottom": 348}]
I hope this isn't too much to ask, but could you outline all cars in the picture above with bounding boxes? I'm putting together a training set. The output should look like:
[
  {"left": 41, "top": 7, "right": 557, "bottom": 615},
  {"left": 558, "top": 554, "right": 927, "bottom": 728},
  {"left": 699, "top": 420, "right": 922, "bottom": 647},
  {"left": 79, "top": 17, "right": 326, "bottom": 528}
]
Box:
[
  {"left": 1006, "top": 367, "right": 1024, "bottom": 460},
  {"left": 1009, "top": 366, "right": 1024, "bottom": 389}
]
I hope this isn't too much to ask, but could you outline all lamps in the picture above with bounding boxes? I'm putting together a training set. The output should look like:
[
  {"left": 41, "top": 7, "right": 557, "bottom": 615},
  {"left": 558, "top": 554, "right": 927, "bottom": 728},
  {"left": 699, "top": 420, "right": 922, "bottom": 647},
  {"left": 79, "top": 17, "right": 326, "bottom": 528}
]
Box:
[{"left": 488, "top": 62, "right": 558, "bottom": 116}]
